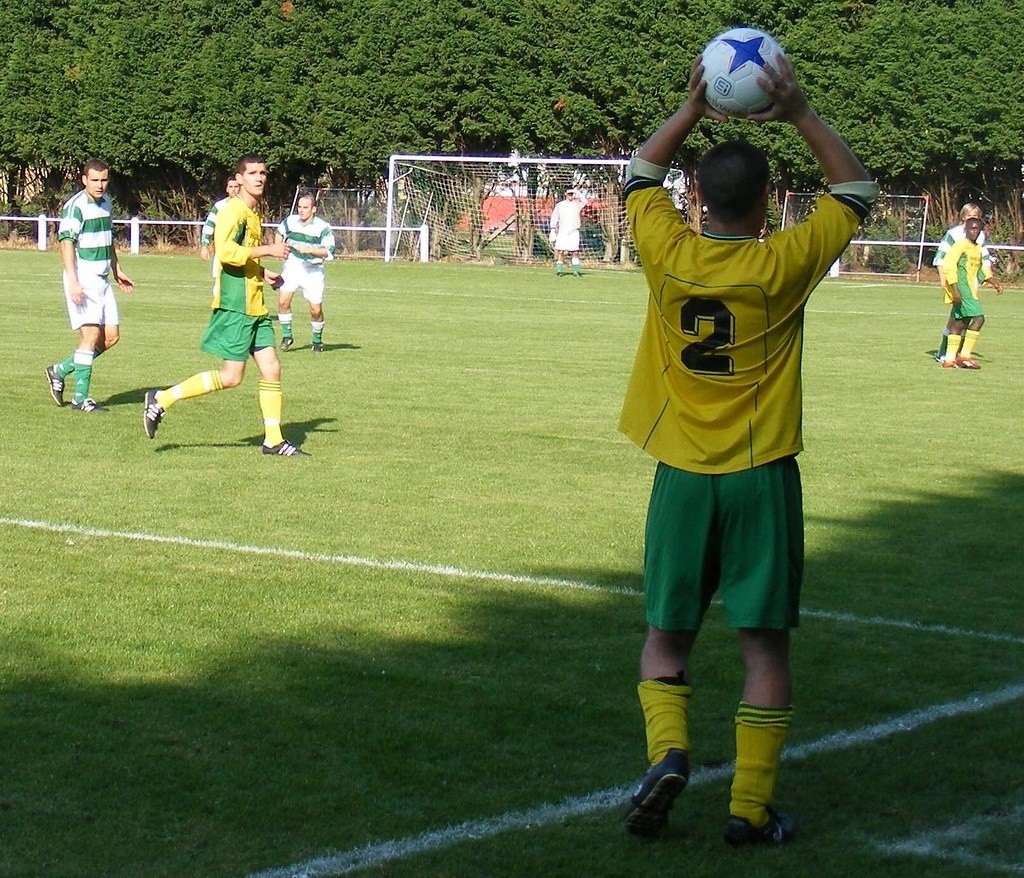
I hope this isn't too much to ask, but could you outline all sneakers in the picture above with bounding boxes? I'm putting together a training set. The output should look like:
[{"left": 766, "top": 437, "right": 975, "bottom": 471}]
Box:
[
  {"left": 45, "top": 363, "right": 67, "bottom": 407},
  {"left": 71, "top": 398, "right": 109, "bottom": 412},
  {"left": 943, "top": 360, "right": 960, "bottom": 369},
  {"left": 279, "top": 337, "right": 294, "bottom": 351},
  {"left": 312, "top": 343, "right": 324, "bottom": 352},
  {"left": 724, "top": 805, "right": 793, "bottom": 849},
  {"left": 143, "top": 389, "right": 167, "bottom": 439},
  {"left": 262, "top": 439, "right": 312, "bottom": 457},
  {"left": 624, "top": 748, "right": 691, "bottom": 836},
  {"left": 954, "top": 356, "right": 981, "bottom": 369}
]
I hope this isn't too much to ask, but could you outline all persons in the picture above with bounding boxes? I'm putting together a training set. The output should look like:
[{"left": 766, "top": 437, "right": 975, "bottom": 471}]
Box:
[
  {"left": 144, "top": 154, "right": 310, "bottom": 456},
  {"left": 274, "top": 193, "right": 335, "bottom": 352},
  {"left": 548, "top": 188, "right": 589, "bottom": 277},
  {"left": 618, "top": 51, "right": 877, "bottom": 846},
  {"left": 200, "top": 175, "right": 240, "bottom": 296},
  {"left": 933, "top": 203, "right": 1003, "bottom": 369},
  {"left": 45, "top": 160, "right": 135, "bottom": 414}
]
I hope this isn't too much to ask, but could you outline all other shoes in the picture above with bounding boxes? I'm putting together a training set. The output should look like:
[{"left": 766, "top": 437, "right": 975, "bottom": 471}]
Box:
[
  {"left": 935, "top": 355, "right": 946, "bottom": 362},
  {"left": 572, "top": 272, "right": 581, "bottom": 277},
  {"left": 557, "top": 272, "right": 563, "bottom": 277}
]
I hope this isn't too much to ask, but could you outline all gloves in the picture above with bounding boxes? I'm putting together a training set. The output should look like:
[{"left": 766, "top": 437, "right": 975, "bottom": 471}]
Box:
[
  {"left": 548, "top": 229, "right": 557, "bottom": 243},
  {"left": 567, "top": 189, "right": 582, "bottom": 199}
]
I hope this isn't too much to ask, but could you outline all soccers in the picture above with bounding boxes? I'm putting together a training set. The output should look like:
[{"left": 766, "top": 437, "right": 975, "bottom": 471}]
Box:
[{"left": 699, "top": 28, "right": 785, "bottom": 118}]
